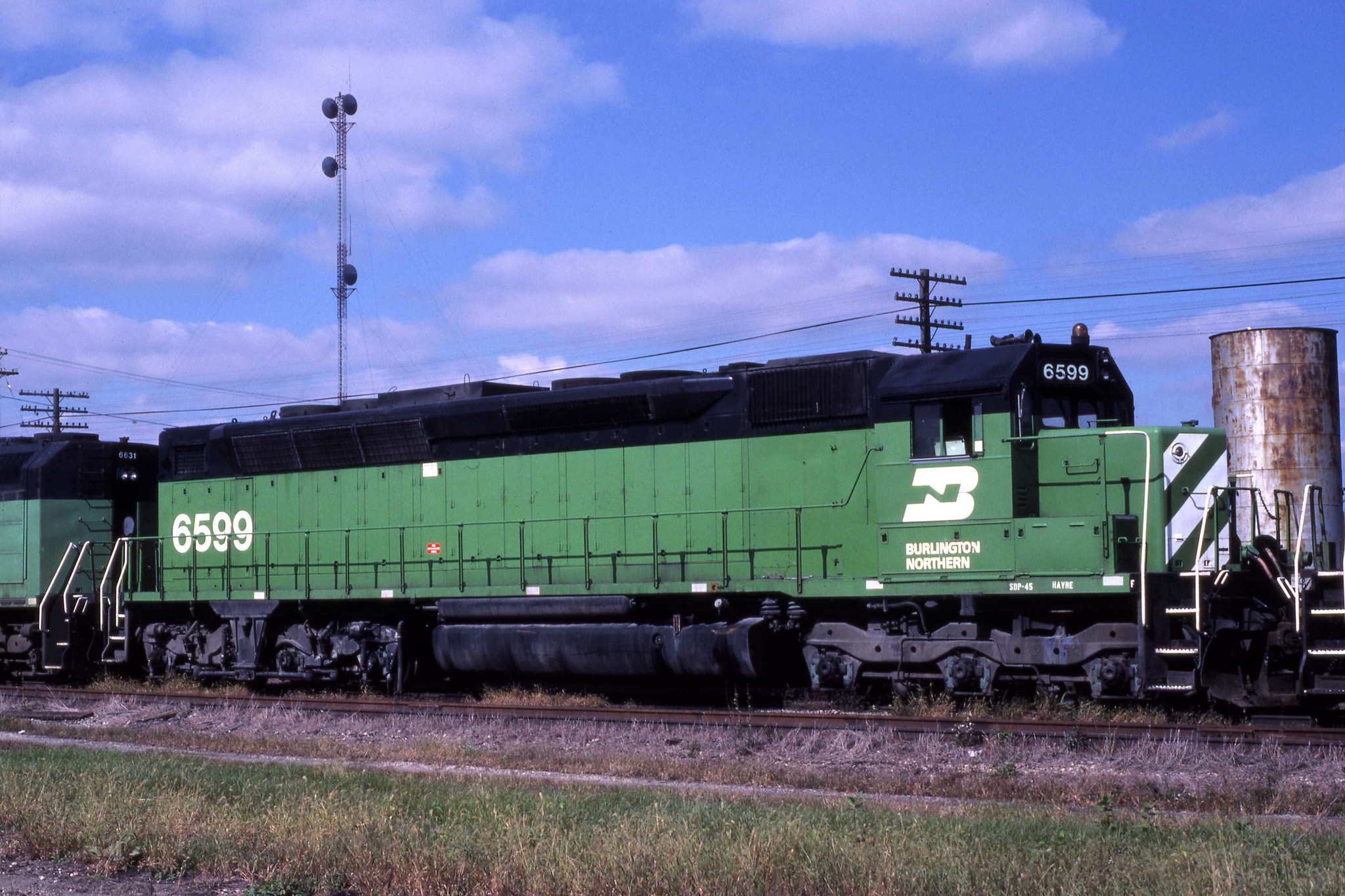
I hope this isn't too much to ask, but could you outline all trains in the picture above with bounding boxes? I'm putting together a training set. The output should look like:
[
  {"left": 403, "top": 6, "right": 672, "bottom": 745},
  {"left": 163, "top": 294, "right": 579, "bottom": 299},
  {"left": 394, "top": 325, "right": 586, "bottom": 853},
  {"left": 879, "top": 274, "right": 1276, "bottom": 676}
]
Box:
[{"left": 0, "top": 320, "right": 1328, "bottom": 710}]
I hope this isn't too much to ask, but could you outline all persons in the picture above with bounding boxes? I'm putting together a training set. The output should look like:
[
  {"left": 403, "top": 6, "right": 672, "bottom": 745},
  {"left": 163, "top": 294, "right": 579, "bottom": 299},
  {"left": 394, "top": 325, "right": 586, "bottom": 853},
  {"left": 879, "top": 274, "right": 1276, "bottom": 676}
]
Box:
[{"left": 1221, "top": 535, "right": 1292, "bottom": 702}]
